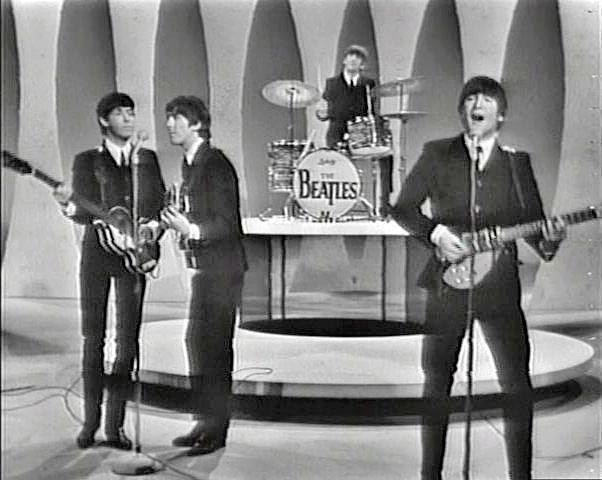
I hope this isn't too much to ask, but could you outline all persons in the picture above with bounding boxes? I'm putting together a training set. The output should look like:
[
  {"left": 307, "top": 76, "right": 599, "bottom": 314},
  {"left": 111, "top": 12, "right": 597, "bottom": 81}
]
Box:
[
  {"left": 391, "top": 75, "right": 567, "bottom": 480},
  {"left": 52, "top": 93, "right": 170, "bottom": 450},
  {"left": 164, "top": 96, "right": 249, "bottom": 455},
  {"left": 321, "top": 45, "right": 376, "bottom": 148}
]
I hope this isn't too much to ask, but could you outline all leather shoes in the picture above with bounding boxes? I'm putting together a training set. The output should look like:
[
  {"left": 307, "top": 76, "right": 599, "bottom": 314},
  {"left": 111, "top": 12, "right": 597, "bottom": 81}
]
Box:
[
  {"left": 172, "top": 429, "right": 225, "bottom": 455},
  {"left": 108, "top": 430, "right": 132, "bottom": 449},
  {"left": 77, "top": 429, "right": 93, "bottom": 446}
]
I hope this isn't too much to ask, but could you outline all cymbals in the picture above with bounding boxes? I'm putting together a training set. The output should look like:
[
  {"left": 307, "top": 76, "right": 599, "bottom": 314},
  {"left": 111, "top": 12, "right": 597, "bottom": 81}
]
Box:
[
  {"left": 262, "top": 79, "right": 320, "bottom": 108},
  {"left": 374, "top": 76, "right": 428, "bottom": 90},
  {"left": 381, "top": 112, "right": 427, "bottom": 118}
]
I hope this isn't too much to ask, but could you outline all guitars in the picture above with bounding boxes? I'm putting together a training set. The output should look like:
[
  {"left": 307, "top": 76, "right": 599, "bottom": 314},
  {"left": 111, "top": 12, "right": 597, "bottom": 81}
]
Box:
[
  {"left": 435, "top": 206, "right": 602, "bottom": 290},
  {"left": 3, "top": 151, "right": 160, "bottom": 274}
]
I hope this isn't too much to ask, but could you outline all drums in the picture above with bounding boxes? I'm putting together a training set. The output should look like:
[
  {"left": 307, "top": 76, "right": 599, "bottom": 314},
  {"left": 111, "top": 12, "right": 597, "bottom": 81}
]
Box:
[
  {"left": 268, "top": 141, "right": 310, "bottom": 193},
  {"left": 293, "top": 147, "right": 363, "bottom": 220},
  {"left": 347, "top": 115, "right": 394, "bottom": 157},
  {"left": 348, "top": 156, "right": 390, "bottom": 216}
]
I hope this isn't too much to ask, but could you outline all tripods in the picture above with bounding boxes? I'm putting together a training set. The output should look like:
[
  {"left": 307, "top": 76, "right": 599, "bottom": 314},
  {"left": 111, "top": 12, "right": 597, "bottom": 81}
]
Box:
[{"left": 258, "top": 85, "right": 407, "bottom": 224}]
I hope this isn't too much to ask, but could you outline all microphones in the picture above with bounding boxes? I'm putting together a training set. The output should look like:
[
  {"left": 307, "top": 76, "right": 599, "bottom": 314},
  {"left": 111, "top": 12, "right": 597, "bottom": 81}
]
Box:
[
  {"left": 131, "top": 129, "right": 149, "bottom": 156},
  {"left": 469, "top": 127, "right": 482, "bottom": 161}
]
